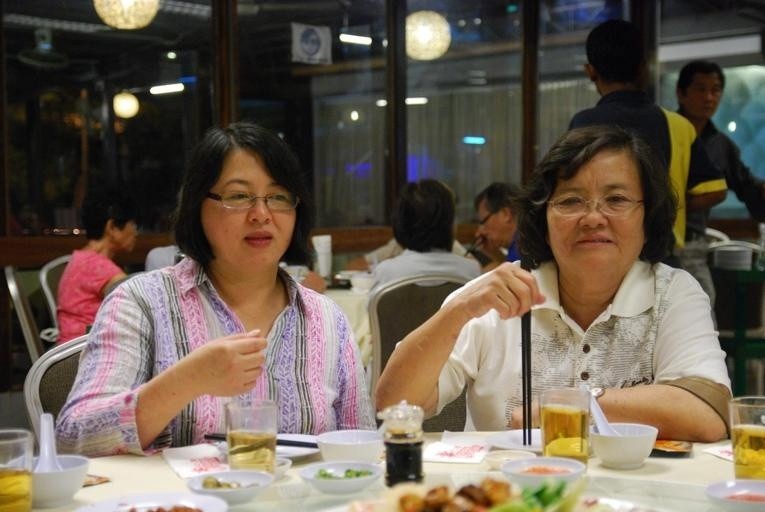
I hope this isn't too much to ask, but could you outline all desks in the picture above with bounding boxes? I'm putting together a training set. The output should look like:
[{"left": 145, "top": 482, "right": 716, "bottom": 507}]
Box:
[{"left": 711, "top": 265, "right": 764, "bottom": 394}]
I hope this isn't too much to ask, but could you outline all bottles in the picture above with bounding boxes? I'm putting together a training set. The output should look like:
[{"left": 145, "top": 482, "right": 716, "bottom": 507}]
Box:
[{"left": 376, "top": 398, "right": 424, "bottom": 484}]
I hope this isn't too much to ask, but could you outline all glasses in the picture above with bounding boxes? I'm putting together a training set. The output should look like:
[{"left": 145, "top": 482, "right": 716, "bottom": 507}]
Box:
[
  {"left": 207, "top": 185, "right": 302, "bottom": 212},
  {"left": 544, "top": 191, "right": 645, "bottom": 217}
]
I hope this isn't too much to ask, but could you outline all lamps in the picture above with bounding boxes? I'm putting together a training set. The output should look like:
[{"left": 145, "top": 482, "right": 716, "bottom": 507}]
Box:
[
  {"left": 93, "top": 0, "right": 159, "bottom": 31},
  {"left": 406, "top": 10, "right": 452, "bottom": 61}
]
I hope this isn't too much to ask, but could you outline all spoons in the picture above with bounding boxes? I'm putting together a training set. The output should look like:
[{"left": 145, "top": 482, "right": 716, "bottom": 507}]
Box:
[
  {"left": 34, "top": 413, "right": 62, "bottom": 471},
  {"left": 591, "top": 396, "right": 618, "bottom": 435}
]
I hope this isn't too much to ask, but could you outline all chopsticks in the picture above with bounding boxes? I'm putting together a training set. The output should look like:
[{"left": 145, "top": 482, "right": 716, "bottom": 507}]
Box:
[
  {"left": 463, "top": 234, "right": 486, "bottom": 256},
  {"left": 203, "top": 432, "right": 317, "bottom": 449},
  {"left": 521, "top": 253, "right": 531, "bottom": 446}
]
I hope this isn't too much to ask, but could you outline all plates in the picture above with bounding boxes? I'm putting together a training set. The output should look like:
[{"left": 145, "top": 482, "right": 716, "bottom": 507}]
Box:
[
  {"left": 73, "top": 492, "right": 228, "bottom": 512},
  {"left": 324, "top": 496, "right": 635, "bottom": 511},
  {"left": 488, "top": 427, "right": 543, "bottom": 453},
  {"left": 275, "top": 433, "right": 319, "bottom": 458},
  {"left": 275, "top": 459, "right": 292, "bottom": 478}
]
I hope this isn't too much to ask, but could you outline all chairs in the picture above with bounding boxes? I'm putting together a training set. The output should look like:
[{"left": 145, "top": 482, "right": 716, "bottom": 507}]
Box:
[
  {"left": 365, "top": 274, "right": 473, "bottom": 431},
  {"left": 5, "top": 265, "right": 45, "bottom": 365},
  {"left": 39, "top": 256, "right": 71, "bottom": 328},
  {"left": 22, "top": 336, "right": 90, "bottom": 434}
]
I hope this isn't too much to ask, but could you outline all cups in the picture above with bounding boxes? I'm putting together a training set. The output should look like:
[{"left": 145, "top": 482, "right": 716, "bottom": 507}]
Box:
[
  {"left": 0, "top": 430, "right": 33, "bottom": 512},
  {"left": 222, "top": 399, "right": 278, "bottom": 470},
  {"left": 727, "top": 396, "right": 765, "bottom": 479},
  {"left": 538, "top": 387, "right": 591, "bottom": 463}
]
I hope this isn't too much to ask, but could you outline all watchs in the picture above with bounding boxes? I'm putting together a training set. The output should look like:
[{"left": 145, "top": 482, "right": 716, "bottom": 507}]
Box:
[{"left": 587, "top": 385, "right": 605, "bottom": 399}]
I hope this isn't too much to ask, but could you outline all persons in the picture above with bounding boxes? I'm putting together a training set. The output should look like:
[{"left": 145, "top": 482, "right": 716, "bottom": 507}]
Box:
[
  {"left": 279, "top": 230, "right": 326, "bottom": 294},
  {"left": 674, "top": 60, "right": 764, "bottom": 312},
  {"left": 370, "top": 177, "right": 482, "bottom": 298},
  {"left": 568, "top": 19, "right": 699, "bottom": 271},
  {"left": 55, "top": 193, "right": 141, "bottom": 345},
  {"left": 54, "top": 122, "right": 377, "bottom": 458},
  {"left": 373, "top": 125, "right": 734, "bottom": 443},
  {"left": 468, "top": 181, "right": 555, "bottom": 273},
  {"left": 343, "top": 235, "right": 482, "bottom": 273}
]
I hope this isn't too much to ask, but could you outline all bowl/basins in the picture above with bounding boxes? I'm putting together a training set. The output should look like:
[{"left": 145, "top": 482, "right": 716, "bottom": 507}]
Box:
[
  {"left": 590, "top": 422, "right": 659, "bottom": 470},
  {"left": 7, "top": 455, "right": 89, "bottom": 507},
  {"left": 299, "top": 461, "right": 380, "bottom": 493},
  {"left": 187, "top": 470, "right": 273, "bottom": 506},
  {"left": 350, "top": 277, "right": 375, "bottom": 290},
  {"left": 705, "top": 480, "right": 764, "bottom": 512},
  {"left": 502, "top": 456, "right": 586, "bottom": 485},
  {"left": 316, "top": 429, "right": 384, "bottom": 463}
]
